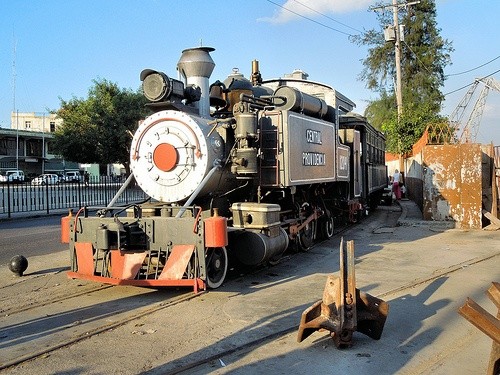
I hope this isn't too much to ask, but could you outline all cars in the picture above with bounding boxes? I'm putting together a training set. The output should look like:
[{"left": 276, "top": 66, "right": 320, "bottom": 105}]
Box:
[
  {"left": 31, "top": 174, "right": 60, "bottom": 186},
  {"left": 0, "top": 174, "right": 8, "bottom": 183}
]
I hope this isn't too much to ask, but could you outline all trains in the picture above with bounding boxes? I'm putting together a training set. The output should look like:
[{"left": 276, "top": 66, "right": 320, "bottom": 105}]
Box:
[{"left": 63, "top": 39, "right": 388, "bottom": 297}]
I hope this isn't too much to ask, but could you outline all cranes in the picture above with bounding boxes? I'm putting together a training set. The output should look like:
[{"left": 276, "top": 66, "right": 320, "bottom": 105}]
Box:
[
  {"left": 447, "top": 78, "right": 499, "bottom": 143},
  {"left": 459, "top": 76, "right": 499, "bottom": 143}
]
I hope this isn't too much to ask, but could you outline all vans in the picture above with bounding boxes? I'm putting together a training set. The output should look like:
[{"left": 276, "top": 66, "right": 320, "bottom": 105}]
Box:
[
  {"left": 65, "top": 171, "right": 82, "bottom": 182},
  {"left": 6, "top": 170, "right": 25, "bottom": 183}
]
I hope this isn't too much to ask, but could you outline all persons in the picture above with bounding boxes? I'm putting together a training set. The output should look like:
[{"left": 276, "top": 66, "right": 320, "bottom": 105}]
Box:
[
  {"left": 392, "top": 169, "right": 403, "bottom": 200},
  {"left": 84, "top": 171, "right": 91, "bottom": 187}
]
